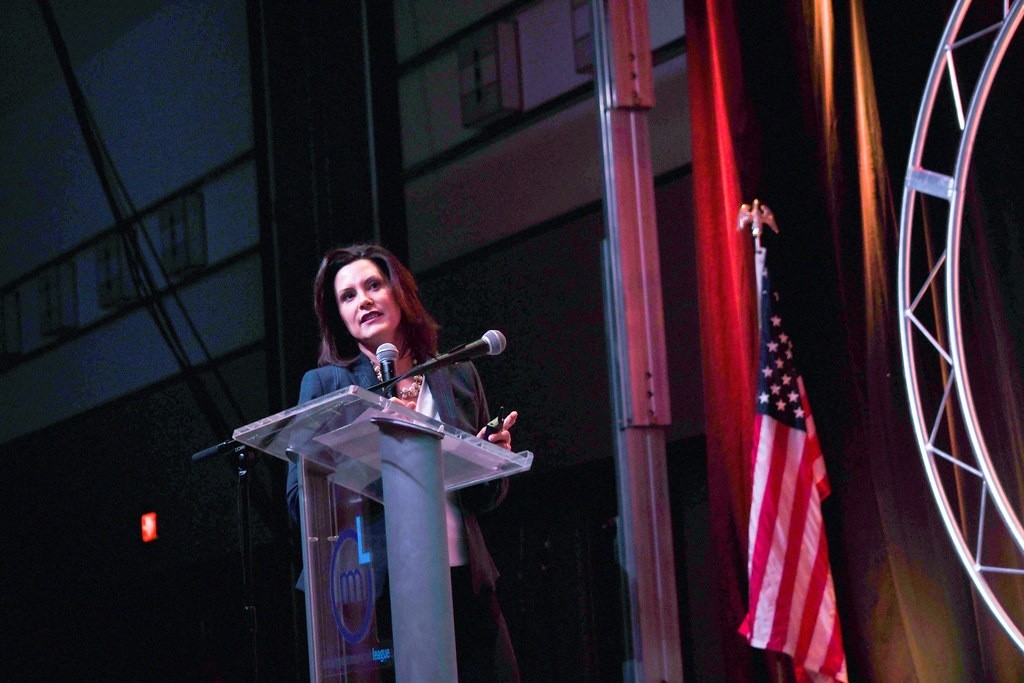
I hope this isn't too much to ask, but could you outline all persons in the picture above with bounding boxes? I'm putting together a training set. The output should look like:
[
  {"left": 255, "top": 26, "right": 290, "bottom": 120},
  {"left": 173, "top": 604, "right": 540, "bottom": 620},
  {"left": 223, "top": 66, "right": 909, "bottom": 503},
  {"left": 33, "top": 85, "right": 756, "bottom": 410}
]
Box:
[{"left": 285, "top": 241, "right": 523, "bottom": 683}]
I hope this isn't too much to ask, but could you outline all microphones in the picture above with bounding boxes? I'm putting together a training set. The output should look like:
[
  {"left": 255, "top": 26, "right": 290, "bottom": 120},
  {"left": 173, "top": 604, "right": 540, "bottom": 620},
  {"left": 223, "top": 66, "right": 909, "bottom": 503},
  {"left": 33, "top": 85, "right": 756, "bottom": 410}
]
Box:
[
  {"left": 411, "top": 329, "right": 507, "bottom": 376},
  {"left": 376, "top": 343, "right": 399, "bottom": 399}
]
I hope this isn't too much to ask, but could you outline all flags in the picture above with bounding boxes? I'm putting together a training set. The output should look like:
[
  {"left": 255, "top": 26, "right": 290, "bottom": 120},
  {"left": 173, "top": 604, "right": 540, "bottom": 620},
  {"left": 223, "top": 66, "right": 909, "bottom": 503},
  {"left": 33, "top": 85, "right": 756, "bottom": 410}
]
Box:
[{"left": 735, "top": 245, "right": 851, "bottom": 683}]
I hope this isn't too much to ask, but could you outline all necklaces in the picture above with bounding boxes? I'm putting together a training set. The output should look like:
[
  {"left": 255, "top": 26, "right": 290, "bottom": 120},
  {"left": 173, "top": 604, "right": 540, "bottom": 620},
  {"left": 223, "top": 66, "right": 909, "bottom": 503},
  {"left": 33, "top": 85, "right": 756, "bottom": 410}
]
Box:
[{"left": 365, "top": 357, "right": 425, "bottom": 400}]
